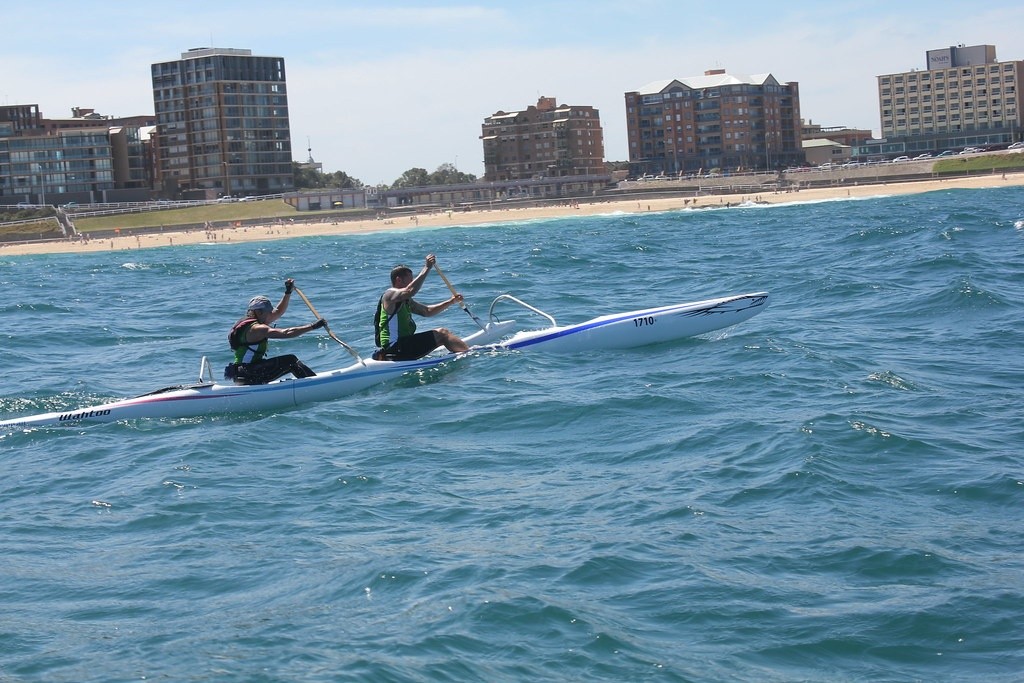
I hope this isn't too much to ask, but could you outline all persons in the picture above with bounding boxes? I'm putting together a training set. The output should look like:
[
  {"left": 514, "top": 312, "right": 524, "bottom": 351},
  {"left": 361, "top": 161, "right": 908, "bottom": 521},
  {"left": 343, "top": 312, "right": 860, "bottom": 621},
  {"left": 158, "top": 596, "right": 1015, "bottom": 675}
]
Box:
[
  {"left": 69, "top": 230, "right": 172, "bottom": 250},
  {"left": 203, "top": 219, "right": 294, "bottom": 240},
  {"left": 720, "top": 183, "right": 812, "bottom": 208},
  {"left": 374, "top": 254, "right": 469, "bottom": 361},
  {"left": 377, "top": 202, "right": 520, "bottom": 226},
  {"left": 684, "top": 198, "right": 697, "bottom": 204},
  {"left": 636, "top": 203, "right": 640, "bottom": 209},
  {"left": 1002, "top": 172, "right": 1005, "bottom": 180},
  {"left": 305, "top": 215, "right": 339, "bottom": 225},
  {"left": 545, "top": 198, "right": 610, "bottom": 209},
  {"left": 228, "top": 278, "right": 328, "bottom": 385}
]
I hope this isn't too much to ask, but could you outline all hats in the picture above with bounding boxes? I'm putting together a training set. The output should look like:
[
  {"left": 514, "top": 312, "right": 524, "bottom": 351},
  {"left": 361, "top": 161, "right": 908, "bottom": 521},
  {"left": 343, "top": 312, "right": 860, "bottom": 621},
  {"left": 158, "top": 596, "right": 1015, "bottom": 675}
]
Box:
[{"left": 248, "top": 296, "right": 279, "bottom": 311}]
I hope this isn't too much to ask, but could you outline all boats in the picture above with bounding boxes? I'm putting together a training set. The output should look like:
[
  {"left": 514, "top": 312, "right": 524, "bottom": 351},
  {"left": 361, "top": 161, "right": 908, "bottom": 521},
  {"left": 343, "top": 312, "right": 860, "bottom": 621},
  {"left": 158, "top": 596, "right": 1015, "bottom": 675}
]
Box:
[{"left": 1, "top": 291, "right": 769, "bottom": 430}]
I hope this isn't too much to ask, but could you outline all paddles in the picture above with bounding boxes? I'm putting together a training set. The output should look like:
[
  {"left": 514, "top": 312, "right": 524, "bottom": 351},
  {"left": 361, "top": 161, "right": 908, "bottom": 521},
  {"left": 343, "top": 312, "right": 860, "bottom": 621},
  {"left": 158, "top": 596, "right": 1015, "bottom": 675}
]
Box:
[
  {"left": 433, "top": 260, "right": 489, "bottom": 336},
  {"left": 293, "top": 284, "right": 367, "bottom": 368}
]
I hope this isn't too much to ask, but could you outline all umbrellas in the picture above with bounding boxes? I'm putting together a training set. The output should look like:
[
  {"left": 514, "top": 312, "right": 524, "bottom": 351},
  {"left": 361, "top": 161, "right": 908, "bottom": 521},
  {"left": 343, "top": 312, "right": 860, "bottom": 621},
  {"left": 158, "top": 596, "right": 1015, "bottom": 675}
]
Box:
[{"left": 334, "top": 202, "right": 342, "bottom": 205}]
